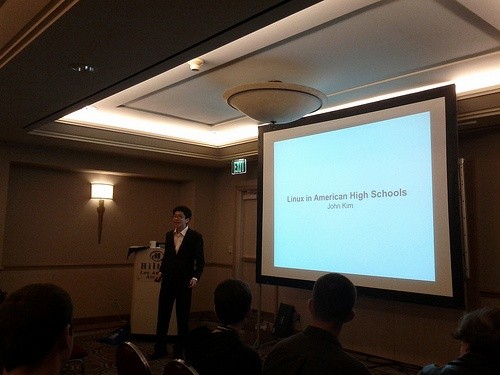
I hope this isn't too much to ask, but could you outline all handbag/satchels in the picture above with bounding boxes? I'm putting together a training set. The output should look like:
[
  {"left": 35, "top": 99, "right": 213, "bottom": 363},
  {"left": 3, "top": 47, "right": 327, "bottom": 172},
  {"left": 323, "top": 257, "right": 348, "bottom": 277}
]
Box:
[{"left": 271, "top": 303, "right": 300, "bottom": 339}]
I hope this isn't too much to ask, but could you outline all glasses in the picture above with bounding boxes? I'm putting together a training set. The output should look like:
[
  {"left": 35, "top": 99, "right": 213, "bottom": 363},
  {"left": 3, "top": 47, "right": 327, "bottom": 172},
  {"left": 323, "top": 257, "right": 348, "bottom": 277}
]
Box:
[{"left": 172, "top": 215, "right": 187, "bottom": 219}]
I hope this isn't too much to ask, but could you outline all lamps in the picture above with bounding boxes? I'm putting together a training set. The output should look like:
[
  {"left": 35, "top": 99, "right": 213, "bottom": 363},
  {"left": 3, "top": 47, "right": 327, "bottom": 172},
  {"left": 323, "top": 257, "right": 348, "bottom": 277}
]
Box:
[
  {"left": 221, "top": 82, "right": 327, "bottom": 125},
  {"left": 90, "top": 182, "right": 114, "bottom": 244}
]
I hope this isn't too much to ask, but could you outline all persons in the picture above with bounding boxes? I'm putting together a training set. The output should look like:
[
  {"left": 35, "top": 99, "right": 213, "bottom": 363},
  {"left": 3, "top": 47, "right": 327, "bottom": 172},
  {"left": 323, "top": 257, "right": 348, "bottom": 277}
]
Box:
[
  {"left": 0, "top": 283, "right": 74, "bottom": 375},
  {"left": 415, "top": 305, "right": 500, "bottom": 375},
  {"left": 148, "top": 206, "right": 205, "bottom": 361},
  {"left": 182, "top": 278, "right": 262, "bottom": 375},
  {"left": 262, "top": 274, "right": 373, "bottom": 375}
]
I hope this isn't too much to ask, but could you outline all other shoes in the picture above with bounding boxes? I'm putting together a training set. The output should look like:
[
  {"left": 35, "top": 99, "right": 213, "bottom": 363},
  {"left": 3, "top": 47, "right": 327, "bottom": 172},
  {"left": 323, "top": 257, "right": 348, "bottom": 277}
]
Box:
[
  {"left": 174, "top": 354, "right": 184, "bottom": 360},
  {"left": 149, "top": 352, "right": 168, "bottom": 361}
]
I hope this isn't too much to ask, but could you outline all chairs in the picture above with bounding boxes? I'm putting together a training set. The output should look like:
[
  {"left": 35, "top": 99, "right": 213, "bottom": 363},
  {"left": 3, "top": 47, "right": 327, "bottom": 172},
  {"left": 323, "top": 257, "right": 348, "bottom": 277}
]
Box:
[{"left": 67, "top": 343, "right": 198, "bottom": 375}]
